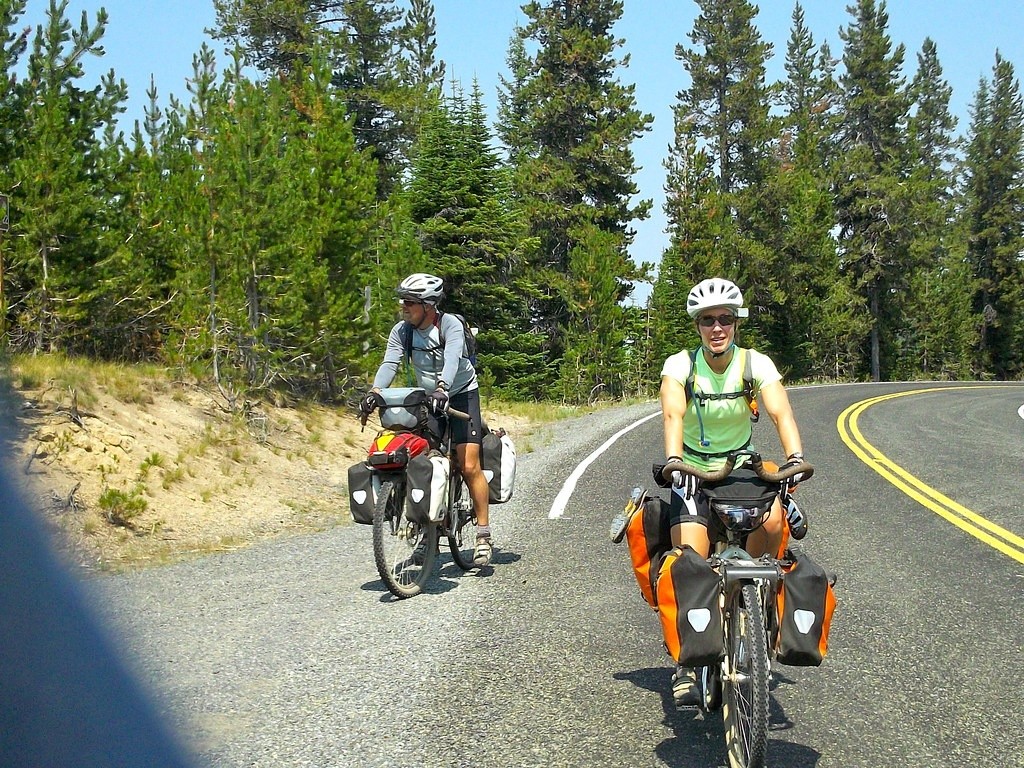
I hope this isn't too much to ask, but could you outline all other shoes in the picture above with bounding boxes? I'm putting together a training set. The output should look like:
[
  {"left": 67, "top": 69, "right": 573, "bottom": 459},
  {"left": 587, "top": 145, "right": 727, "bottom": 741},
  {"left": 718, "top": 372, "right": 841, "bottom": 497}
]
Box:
[
  {"left": 411, "top": 539, "right": 440, "bottom": 564},
  {"left": 670, "top": 667, "right": 701, "bottom": 707},
  {"left": 784, "top": 494, "right": 808, "bottom": 540},
  {"left": 609, "top": 487, "right": 648, "bottom": 543},
  {"left": 750, "top": 648, "right": 773, "bottom": 681},
  {"left": 473, "top": 533, "right": 494, "bottom": 566}
]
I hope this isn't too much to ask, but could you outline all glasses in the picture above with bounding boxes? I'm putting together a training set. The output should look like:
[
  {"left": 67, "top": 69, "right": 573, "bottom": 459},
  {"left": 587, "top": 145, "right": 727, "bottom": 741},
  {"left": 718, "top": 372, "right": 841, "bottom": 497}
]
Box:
[
  {"left": 404, "top": 300, "right": 426, "bottom": 306},
  {"left": 697, "top": 313, "right": 734, "bottom": 326}
]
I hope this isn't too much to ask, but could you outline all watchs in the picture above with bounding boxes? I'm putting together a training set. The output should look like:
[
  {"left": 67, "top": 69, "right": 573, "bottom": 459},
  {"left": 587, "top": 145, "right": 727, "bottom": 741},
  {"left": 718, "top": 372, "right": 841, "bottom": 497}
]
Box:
[
  {"left": 437, "top": 384, "right": 450, "bottom": 392},
  {"left": 787, "top": 452, "right": 803, "bottom": 460}
]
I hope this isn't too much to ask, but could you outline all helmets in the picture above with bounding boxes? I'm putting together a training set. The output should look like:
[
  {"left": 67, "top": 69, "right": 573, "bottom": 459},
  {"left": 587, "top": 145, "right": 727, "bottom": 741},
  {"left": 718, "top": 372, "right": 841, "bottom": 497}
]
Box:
[
  {"left": 686, "top": 278, "right": 749, "bottom": 319},
  {"left": 397, "top": 273, "right": 446, "bottom": 305}
]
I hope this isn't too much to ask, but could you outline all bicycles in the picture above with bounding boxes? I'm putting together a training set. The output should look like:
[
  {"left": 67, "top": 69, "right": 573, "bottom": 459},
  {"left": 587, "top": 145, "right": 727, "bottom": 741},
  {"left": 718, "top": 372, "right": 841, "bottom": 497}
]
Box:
[
  {"left": 661, "top": 449, "right": 814, "bottom": 768},
  {"left": 354, "top": 389, "right": 491, "bottom": 598}
]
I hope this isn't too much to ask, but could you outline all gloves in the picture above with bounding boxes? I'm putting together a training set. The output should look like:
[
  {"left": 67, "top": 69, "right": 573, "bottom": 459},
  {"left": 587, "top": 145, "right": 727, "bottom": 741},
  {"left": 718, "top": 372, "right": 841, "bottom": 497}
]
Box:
[
  {"left": 666, "top": 456, "right": 700, "bottom": 500},
  {"left": 358, "top": 391, "right": 376, "bottom": 425},
  {"left": 427, "top": 386, "right": 449, "bottom": 417},
  {"left": 779, "top": 452, "right": 804, "bottom": 500}
]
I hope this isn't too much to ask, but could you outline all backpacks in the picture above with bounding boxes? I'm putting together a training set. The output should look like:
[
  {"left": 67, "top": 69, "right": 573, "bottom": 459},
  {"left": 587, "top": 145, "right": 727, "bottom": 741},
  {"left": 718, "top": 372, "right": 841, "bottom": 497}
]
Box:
[{"left": 405, "top": 311, "right": 478, "bottom": 367}]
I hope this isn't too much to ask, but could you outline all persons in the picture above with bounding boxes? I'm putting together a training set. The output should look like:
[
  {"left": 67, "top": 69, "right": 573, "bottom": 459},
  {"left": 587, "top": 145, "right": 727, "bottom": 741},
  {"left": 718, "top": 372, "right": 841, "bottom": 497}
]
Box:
[
  {"left": 358, "top": 273, "right": 494, "bottom": 565},
  {"left": 659, "top": 278, "right": 804, "bottom": 705}
]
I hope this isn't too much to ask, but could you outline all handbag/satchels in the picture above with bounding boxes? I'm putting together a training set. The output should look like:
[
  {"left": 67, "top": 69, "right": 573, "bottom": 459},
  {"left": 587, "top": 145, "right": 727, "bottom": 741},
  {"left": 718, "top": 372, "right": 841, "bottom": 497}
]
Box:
[
  {"left": 771, "top": 548, "right": 837, "bottom": 668},
  {"left": 406, "top": 450, "right": 453, "bottom": 522},
  {"left": 477, "top": 428, "right": 517, "bottom": 504},
  {"left": 654, "top": 545, "right": 723, "bottom": 665},
  {"left": 625, "top": 495, "right": 672, "bottom": 613},
  {"left": 348, "top": 462, "right": 402, "bottom": 525}
]
